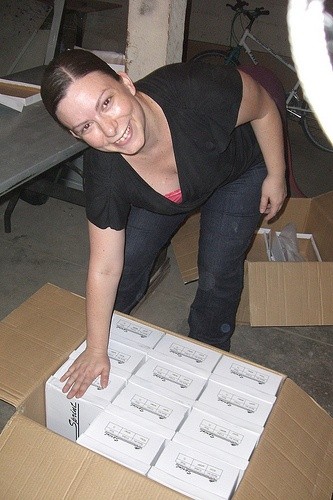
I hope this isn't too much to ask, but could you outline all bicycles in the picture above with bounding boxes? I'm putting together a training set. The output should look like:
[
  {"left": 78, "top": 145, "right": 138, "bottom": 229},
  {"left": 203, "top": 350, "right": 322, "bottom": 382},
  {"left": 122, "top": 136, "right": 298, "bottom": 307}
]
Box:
[{"left": 191, "top": 0, "right": 333, "bottom": 155}]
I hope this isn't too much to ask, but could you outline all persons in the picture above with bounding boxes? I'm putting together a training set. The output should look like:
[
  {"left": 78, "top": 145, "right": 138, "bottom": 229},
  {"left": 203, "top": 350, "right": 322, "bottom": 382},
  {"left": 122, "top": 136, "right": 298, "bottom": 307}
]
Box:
[{"left": 37, "top": 47, "right": 288, "bottom": 399}]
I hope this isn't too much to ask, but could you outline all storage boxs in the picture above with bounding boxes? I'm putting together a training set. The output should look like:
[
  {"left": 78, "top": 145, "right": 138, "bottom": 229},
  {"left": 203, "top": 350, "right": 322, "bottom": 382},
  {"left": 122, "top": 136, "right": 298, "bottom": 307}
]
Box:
[
  {"left": 0, "top": 281, "right": 333, "bottom": 500},
  {"left": 171, "top": 189, "right": 333, "bottom": 327}
]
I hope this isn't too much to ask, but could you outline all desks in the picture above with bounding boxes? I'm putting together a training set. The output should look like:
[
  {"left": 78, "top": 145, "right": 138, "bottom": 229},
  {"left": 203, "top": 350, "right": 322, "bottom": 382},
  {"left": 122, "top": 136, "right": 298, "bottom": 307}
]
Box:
[{"left": 0, "top": 100, "right": 89, "bottom": 234}]
